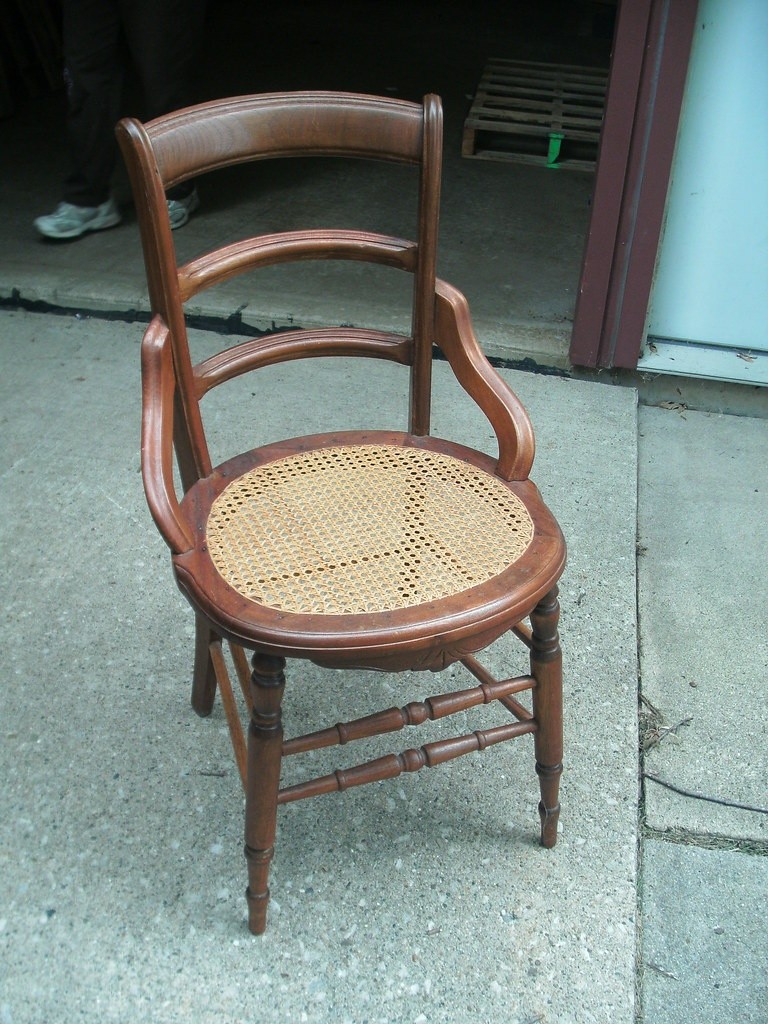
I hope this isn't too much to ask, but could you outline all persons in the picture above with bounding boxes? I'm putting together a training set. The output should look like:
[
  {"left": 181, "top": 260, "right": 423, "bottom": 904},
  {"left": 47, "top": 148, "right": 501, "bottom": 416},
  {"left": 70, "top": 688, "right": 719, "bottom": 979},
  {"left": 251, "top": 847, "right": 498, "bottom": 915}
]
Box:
[{"left": 33, "top": 0, "right": 205, "bottom": 238}]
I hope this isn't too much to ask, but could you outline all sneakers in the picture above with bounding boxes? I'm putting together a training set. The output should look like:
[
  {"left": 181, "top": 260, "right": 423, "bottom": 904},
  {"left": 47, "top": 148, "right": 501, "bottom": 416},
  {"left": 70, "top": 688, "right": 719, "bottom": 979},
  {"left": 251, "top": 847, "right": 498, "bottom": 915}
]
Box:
[
  {"left": 166, "top": 178, "right": 204, "bottom": 229},
  {"left": 34, "top": 193, "right": 121, "bottom": 238}
]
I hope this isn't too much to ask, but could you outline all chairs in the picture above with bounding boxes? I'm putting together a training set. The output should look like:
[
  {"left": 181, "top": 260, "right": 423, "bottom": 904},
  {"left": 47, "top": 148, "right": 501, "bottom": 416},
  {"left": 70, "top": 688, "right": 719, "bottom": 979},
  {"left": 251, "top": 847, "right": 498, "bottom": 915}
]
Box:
[{"left": 117, "top": 93, "right": 566, "bottom": 937}]
